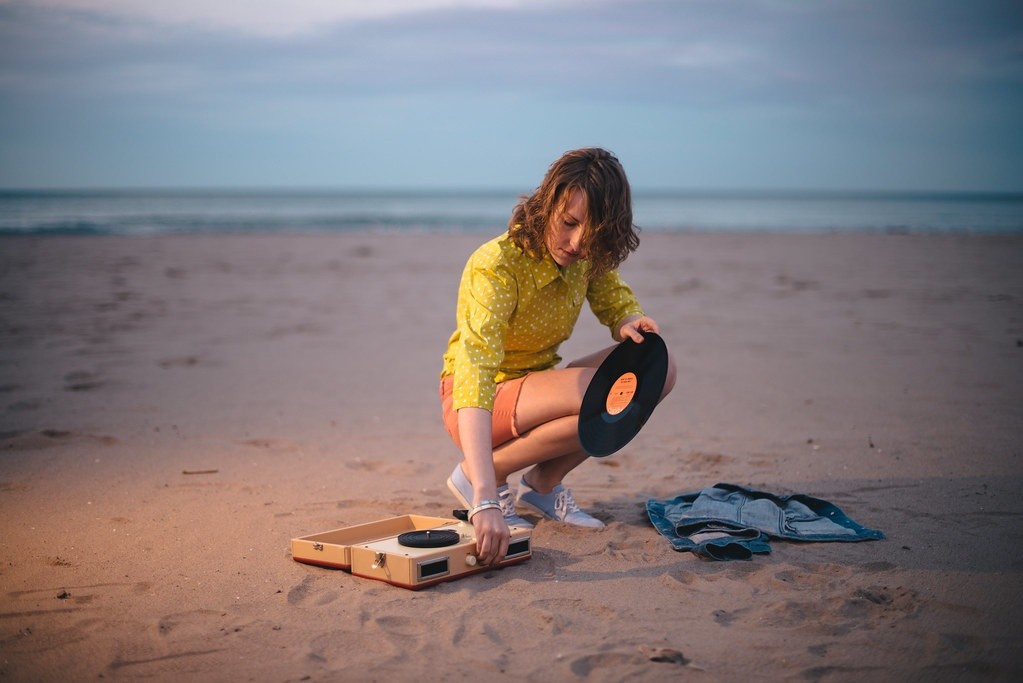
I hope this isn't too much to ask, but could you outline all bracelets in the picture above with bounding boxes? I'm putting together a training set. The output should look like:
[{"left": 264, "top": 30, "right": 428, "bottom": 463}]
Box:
[{"left": 467, "top": 499, "right": 503, "bottom": 524}]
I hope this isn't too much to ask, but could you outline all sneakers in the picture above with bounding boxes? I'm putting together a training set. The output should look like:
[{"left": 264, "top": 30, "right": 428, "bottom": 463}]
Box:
[
  {"left": 446, "top": 463, "right": 534, "bottom": 530},
  {"left": 516, "top": 474, "right": 606, "bottom": 528}
]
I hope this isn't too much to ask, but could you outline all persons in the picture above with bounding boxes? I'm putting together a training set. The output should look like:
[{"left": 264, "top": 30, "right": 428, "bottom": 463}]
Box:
[{"left": 438, "top": 146, "right": 677, "bottom": 566}]
